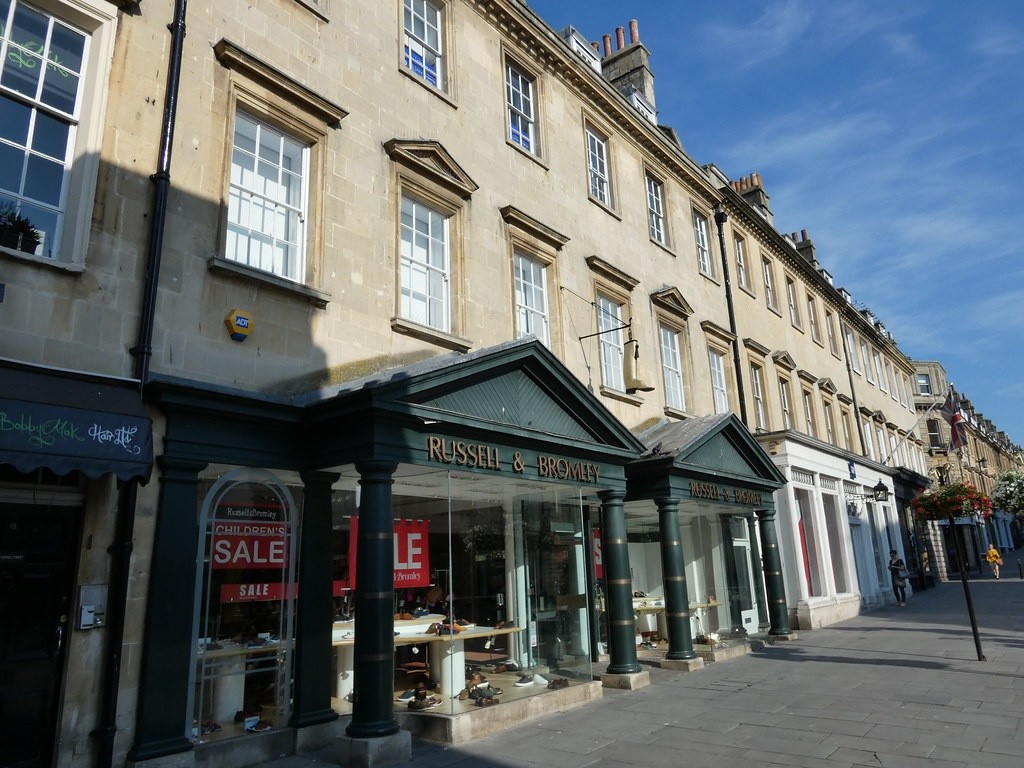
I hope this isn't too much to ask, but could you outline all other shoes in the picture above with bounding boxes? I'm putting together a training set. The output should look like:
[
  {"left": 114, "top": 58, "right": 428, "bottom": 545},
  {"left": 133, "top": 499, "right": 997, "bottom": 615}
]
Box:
[
  {"left": 547, "top": 678, "right": 569, "bottom": 689},
  {"left": 901, "top": 602, "right": 905, "bottom": 606},
  {"left": 532, "top": 674, "right": 548, "bottom": 685},
  {"left": 394, "top": 604, "right": 504, "bottom": 711},
  {"left": 191, "top": 631, "right": 280, "bottom": 733},
  {"left": 493, "top": 664, "right": 506, "bottom": 673},
  {"left": 495, "top": 620, "right": 515, "bottom": 629},
  {"left": 997, "top": 578, "right": 998, "bottom": 580},
  {"left": 635, "top": 591, "right": 667, "bottom": 649},
  {"left": 342, "top": 632, "right": 355, "bottom": 639},
  {"left": 506, "top": 663, "right": 519, "bottom": 672},
  {"left": 513, "top": 675, "right": 534, "bottom": 686},
  {"left": 994, "top": 575, "right": 996, "bottom": 578},
  {"left": 896, "top": 602, "right": 901, "bottom": 605}
]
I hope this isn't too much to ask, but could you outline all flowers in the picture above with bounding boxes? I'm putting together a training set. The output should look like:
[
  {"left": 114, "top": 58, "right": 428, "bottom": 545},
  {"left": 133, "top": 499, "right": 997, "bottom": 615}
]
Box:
[{"left": 911, "top": 478, "right": 994, "bottom": 524}]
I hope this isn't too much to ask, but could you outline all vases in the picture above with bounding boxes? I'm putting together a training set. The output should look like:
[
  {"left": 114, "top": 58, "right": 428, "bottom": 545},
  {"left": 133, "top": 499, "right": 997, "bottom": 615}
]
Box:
[{"left": 934, "top": 499, "right": 964, "bottom": 520}]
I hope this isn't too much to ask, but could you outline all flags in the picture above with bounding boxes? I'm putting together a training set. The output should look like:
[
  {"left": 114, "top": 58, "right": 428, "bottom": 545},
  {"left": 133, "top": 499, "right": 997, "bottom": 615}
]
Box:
[{"left": 934, "top": 382, "right": 968, "bottom": 454}]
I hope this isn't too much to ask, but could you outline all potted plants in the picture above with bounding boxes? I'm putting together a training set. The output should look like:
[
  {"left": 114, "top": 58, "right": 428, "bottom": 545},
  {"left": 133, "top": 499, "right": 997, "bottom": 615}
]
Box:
[
  {"left": 0, "top": 200, "right": 37, "bottom": 250},
  {"left": 22, "top": 229, "right": 41, "bottom": 255}
]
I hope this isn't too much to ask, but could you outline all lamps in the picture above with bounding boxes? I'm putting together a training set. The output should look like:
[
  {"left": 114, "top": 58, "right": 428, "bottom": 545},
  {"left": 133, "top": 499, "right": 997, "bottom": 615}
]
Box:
[{"left": 873, "top": 478, "right": 890, "bottom": 502}]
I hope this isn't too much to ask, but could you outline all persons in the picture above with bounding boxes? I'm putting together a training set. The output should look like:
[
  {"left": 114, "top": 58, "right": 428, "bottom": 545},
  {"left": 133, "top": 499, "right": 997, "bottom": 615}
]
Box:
[
  {"left": 888, "top": 550, "right": 906, "bottom": 607},
  {"left": 986, "top": 544, "right": 1000, "bottom": 580}
]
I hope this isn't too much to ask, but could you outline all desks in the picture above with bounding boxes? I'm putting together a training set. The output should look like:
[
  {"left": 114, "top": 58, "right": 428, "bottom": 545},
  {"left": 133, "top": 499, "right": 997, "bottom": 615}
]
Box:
[
  {"left": 332, "top": 614, "right": 524, "bottom": 698},
  {"left": 197, "top": 642, "right": 294, "bottom": 721},
  {"left": 633, "top": 595, "right": 722, "bottom": 641}
]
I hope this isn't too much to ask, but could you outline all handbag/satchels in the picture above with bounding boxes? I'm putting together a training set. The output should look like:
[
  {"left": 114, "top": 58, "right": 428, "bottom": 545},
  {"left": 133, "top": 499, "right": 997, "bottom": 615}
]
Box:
[
  {"left": 996, "top": 558, "right": 1002, "bottom": 566},
  {"left": 898, "top": 559, "right": 909, "bottom": 578}
]
